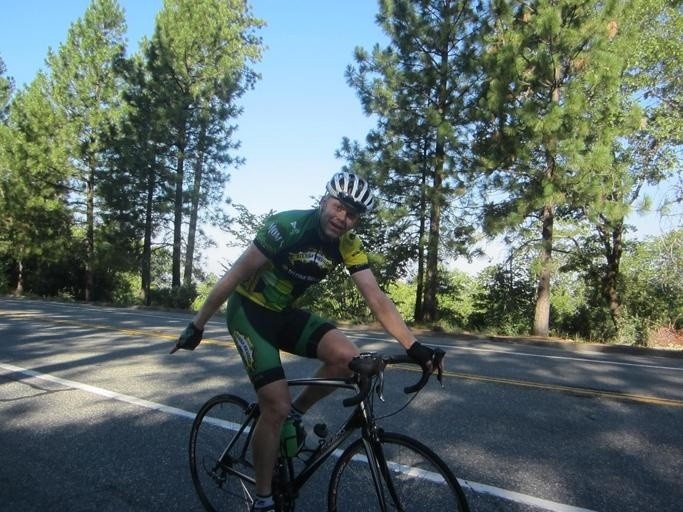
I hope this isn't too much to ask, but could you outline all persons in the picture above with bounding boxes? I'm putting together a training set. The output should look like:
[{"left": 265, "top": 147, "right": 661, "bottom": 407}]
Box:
[{"left": 168, "top": 172, "right": 444, "bottom": 512}]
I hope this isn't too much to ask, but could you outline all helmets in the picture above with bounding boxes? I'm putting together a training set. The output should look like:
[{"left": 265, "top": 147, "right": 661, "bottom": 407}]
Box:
[{"left": 326, "top": 172, "right": 374, "bottom": 213}]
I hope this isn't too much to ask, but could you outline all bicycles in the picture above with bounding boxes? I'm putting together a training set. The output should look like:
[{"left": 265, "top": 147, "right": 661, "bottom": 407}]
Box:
[{"left": 189, "top": 345, "right": 469, "bottom": 512}]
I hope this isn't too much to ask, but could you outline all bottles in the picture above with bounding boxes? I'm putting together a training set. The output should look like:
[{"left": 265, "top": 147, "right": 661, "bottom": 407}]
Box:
[{"left": 279, "top": 417, "right": 297, "bottom": 459}]
